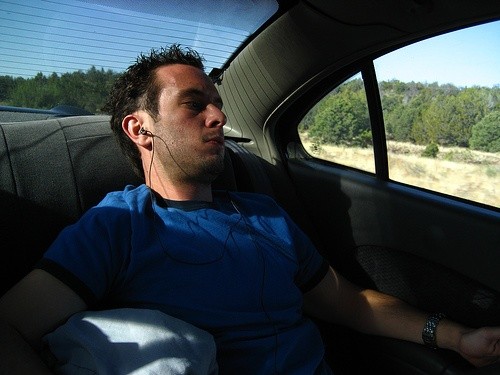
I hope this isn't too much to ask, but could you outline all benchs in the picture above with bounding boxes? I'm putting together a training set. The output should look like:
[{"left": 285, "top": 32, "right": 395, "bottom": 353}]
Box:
[{"left": 0, "top": 115, "right": 294, "bottom": 375}]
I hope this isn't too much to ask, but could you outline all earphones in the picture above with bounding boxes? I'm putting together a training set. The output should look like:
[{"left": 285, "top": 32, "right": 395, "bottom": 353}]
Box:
[{"left": 140, "top": 127, "right": 152, "bottom": 135}]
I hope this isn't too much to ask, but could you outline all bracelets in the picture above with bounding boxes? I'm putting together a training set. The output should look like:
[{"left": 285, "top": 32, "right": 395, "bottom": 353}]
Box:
[{"left": 422, "top": 312, "right": 446, "bottom": 350}]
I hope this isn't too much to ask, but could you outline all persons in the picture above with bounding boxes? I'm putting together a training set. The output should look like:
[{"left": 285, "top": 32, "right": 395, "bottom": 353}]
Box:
[{"left": 0, "top": 44, "right": 500, "bottom": 375}]
